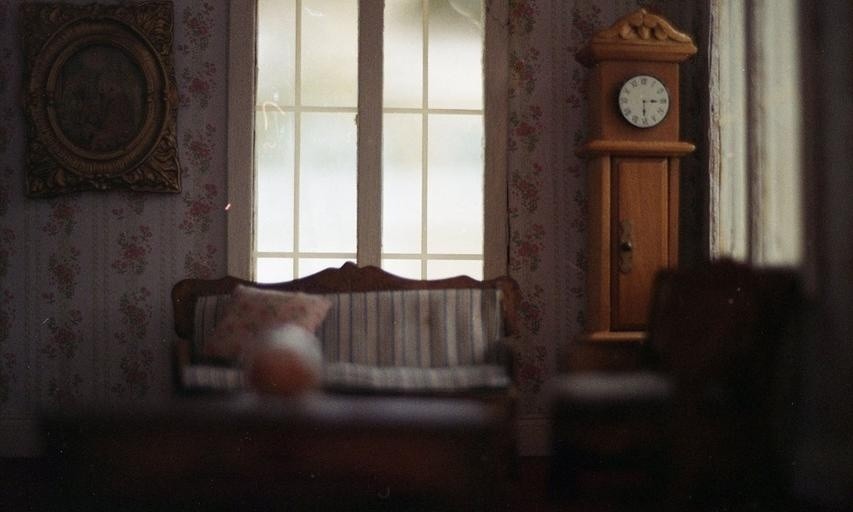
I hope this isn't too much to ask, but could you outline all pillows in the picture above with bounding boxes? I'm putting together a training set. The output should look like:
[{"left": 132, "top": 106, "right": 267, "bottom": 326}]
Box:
[{"left": 197, "top": 280, "right": 330, "bottom": 368}]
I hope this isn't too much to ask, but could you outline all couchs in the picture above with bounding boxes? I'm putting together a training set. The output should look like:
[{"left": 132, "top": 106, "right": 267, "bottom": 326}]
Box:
[{"left": 168, "top": 260, "right": 520, "bottom": 404}]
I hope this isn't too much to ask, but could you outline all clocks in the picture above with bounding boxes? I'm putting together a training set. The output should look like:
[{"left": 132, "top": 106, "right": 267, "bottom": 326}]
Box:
[{"left": 561, "top": 9, "right": 697, "bottom": 372}]
